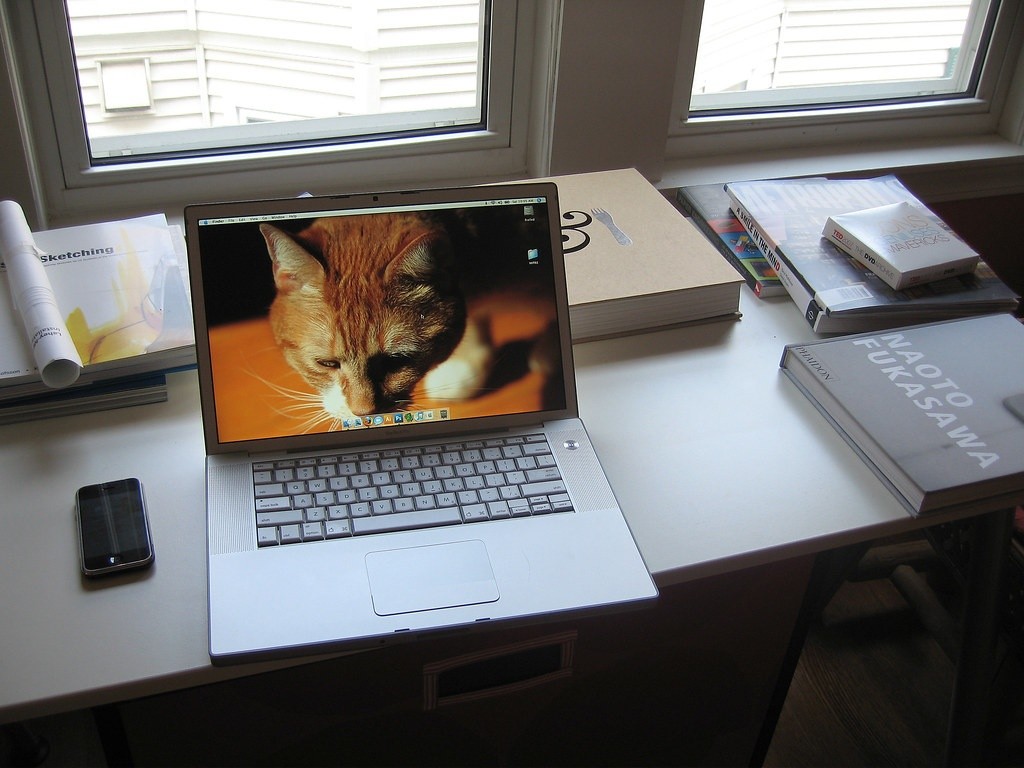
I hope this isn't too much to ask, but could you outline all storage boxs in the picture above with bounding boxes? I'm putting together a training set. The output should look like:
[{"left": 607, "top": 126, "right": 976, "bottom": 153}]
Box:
[{"left": 822, "top": 202, "right": 978, "bottom": 290}]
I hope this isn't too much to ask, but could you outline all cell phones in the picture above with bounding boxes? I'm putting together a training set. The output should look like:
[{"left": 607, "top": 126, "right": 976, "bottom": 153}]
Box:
[{"left": 76, "top": 477, "right": 156, "bottom": 577}]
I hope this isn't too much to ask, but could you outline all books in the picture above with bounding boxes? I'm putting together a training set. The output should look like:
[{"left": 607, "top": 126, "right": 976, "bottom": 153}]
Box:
[
  {"left": 0, "top": 200, "right": 197, "bottom": 427},
  {"left": 464, "top": 166, "right": 747, "bottom": 345},
  {"left": 676, "top": 173, "right": 1024, "bottom": 516}
]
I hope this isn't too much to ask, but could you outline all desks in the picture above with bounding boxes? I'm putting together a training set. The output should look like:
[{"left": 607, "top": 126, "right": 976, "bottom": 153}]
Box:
[{"left": 0, "top": 219, "right": 1024, "bottom": 768}]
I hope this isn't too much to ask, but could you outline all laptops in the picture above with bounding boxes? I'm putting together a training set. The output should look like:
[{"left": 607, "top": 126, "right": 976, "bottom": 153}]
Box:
[{"left": 184, "top": 182, "right": 663, "bottom": 668}]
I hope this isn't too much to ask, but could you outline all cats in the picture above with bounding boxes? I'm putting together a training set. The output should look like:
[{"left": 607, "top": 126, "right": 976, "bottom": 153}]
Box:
[{"left": 246, "top": 202, "right": 566, "bottom": 435}]
